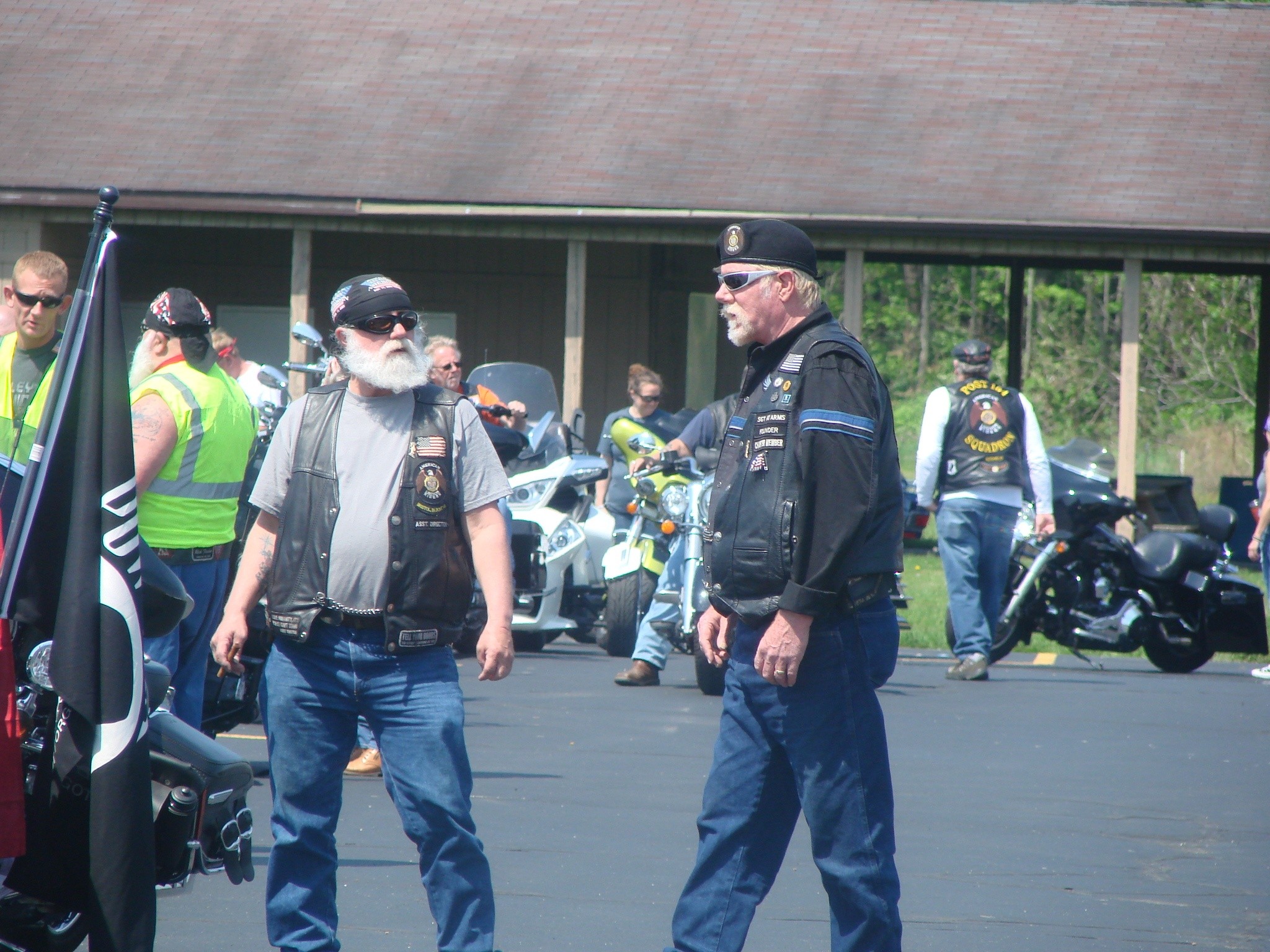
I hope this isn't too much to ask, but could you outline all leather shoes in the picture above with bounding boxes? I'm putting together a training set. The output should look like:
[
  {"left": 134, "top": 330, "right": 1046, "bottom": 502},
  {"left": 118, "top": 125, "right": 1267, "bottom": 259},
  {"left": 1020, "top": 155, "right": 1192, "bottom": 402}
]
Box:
[
  {"left": 614, "top": 659, "right": 662, "bottom": 686},
  {"left": 343, "top": 746, "right": 383, "bottom": 778}
]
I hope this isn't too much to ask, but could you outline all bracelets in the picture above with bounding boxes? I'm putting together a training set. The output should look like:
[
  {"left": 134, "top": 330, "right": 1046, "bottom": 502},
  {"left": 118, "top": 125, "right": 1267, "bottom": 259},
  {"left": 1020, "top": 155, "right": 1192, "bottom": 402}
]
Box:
[{"left": 1252, "top": 535, "right": 1262, "bottom": 543}]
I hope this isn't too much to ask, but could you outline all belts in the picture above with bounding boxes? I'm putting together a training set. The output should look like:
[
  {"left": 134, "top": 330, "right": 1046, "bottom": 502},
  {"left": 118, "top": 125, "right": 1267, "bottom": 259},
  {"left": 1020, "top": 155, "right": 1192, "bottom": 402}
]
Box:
[{"left": 320, "top": 607, "right": 385, "bottom": 631}]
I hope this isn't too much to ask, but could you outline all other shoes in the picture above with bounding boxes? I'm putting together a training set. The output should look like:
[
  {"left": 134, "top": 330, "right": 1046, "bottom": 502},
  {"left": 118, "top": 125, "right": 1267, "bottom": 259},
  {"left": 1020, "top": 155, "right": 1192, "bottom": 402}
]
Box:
[
  {"left": 1250, "top": 663, "right": 1270, "bottom": 679},
  {"left": 946, "top": 653, "right": 989, "bottom": 681}
]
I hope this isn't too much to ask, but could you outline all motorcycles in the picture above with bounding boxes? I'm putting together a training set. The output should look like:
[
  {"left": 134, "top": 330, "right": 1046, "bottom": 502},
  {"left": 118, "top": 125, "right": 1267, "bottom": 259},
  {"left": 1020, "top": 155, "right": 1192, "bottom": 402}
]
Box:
[
  {"left": 0, "top": 629, "right": 255, "bottom": 952},
  {"left": 941, "top": 434, "right": 1241, "bottom": 675},
  {"left": 282, "top": 319, "right": 729, "bottom": 698}
]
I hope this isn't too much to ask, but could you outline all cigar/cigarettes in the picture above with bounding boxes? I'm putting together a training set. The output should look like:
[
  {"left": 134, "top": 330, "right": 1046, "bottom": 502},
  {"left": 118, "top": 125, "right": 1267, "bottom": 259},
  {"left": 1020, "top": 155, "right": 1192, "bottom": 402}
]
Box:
[{"left": 217, "top": 643, "right": 241, "bottom": 678}]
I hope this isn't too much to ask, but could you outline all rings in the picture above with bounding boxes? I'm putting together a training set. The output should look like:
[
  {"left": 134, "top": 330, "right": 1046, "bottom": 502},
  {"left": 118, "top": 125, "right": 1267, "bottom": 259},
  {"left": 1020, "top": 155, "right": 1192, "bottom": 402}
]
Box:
[{"left": 774, "top": 669, "right": 785, "bottom": 676}]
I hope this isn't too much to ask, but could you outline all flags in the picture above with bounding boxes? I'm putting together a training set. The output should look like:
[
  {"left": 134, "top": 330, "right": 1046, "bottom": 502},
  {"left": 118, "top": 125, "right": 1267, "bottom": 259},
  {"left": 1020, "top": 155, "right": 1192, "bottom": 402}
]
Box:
[{"left": 0, "top": 228, "right": 160, "bottom": 952}]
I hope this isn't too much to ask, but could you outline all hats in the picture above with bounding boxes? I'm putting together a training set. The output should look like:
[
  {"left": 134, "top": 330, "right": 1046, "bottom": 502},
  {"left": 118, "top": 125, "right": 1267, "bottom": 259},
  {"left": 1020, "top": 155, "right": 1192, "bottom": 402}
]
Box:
[
  {"left": 951, "top": 340, "right": 990, "bottom": 365},
  {"left": 141, "top": 287, "right": 212, "bottom": 337},
  {"left": 717, "top": 218, "right": 823, "bottom": 276},
  {"left": 1263, "top": 416, "right": 1270, "bottom": 432},
  {"left": 330, "top": 273, "right": 413, "bottom": 325}
]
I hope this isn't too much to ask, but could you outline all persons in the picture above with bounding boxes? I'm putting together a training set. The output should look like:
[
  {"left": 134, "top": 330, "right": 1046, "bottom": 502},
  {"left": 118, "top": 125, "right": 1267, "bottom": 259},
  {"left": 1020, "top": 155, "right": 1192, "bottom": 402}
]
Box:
[
  {"left": 125, "top": 285, "right": 288, "bottom": 743},
  {"left": 916, "top": 338, "right": 1056, "bottom": 686},
  {"left": 0, "top": 250, "right": 75, "bottom": 558},
  {"left": 208, "top": 274, "right": 528, "bottom": 952},
  {"left": 1248, "top": 409, "right": 1270, "bottom": 680},
  {"left": 667, "top": 218, "right": 906, "bottom": 952},
  {"left": 614, "top": 392, "right": 740, "bottom": 686},
  {"left": 597, "top": 362, "right": 678, "bottom": 554}
]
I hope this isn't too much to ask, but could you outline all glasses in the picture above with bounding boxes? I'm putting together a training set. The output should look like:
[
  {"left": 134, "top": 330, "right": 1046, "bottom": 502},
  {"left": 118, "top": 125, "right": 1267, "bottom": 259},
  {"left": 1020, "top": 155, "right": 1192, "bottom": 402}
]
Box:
[
  {"left": 139, "top": 322, "right": 149, "bottom": 333},
  {"left": 637, "top": 392, "right": 661, "bottom": 403},
  {"left": 346, "top": 310, "right": 419, "bottom": 335},
  {"left": 13, "top": 289, "right": 61, "bottom": 309},
  {"left": 1263, "top": 430, "right": 1270, "bottom": 435},
  {"left": 434, "top": 361, "right": 461, "bottom": 370},
  {"left": 716, "top": 270, "right": 776, "bottom": 292}
]
